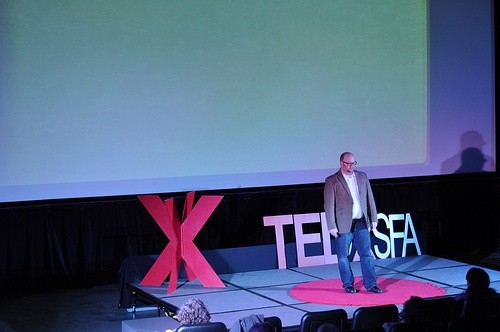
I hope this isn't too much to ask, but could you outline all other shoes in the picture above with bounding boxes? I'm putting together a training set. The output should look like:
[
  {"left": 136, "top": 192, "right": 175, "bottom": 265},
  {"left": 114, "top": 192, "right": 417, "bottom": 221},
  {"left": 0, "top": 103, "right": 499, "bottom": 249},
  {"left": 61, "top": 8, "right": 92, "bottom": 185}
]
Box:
[
  {"left": 371, "top": 286, "right": 382, "bottom": 293},
  {"left": 345, "top": 286, "right": 356, "bottom": 293}
]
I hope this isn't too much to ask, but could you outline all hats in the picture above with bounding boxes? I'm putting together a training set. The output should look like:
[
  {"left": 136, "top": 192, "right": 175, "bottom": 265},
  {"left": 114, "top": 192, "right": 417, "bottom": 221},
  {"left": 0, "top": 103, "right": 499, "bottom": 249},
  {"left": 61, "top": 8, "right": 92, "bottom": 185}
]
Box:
[{"left": 177, "top": 299, "right": 211, "bottom": 325}]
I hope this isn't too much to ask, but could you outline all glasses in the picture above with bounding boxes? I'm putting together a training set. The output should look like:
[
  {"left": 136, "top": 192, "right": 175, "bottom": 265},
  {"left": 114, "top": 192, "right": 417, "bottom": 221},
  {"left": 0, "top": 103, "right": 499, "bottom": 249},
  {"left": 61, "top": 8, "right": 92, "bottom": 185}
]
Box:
[{"left": 342, "top": 161, "right": 357, "bottom": 166}]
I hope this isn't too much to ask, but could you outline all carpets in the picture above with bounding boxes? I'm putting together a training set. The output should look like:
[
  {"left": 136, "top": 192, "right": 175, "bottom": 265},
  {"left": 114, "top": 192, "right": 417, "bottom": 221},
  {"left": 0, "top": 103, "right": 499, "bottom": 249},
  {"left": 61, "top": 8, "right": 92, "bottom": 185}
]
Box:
[{"left": 289, "top": 277, "right": 446, "bottom": 306}]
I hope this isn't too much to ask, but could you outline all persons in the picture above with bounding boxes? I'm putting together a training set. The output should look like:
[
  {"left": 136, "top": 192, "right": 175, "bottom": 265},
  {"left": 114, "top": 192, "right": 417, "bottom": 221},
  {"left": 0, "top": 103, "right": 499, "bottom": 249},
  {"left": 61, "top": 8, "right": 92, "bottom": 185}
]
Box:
[
  {"left": 382, "top": 296, "right": 424, "bottom": 332},
  {"left": 448, "top": 267, "right": 498, "bottom": 332},
  {"left": 165, "top": 298, "right": 211, "bottom": 332},
  {"left": 316, "top": 322, "right": 340, "bottom": 332},
  {"left": 248, "top": 323, "right": 275, "bottom": 332},
  {"left": 324, "top": 152, "right": 383, "bottom": 294}
]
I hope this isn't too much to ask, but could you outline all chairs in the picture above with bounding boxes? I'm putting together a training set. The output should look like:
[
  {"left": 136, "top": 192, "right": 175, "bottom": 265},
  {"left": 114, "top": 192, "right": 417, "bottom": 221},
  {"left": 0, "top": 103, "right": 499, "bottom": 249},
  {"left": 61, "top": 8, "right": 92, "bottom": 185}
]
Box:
[
  {"left": 234, "top": 317, "right": 282, "bottom": 332},
  {"left": 299, "top": 309, "right": 348, "bottom": 332},
  {"left": 351, "top": 304, "right": 399, "bottom": 332},
  {"left": 176, "top": 322, "right": 227, "bottom": 332}
]
